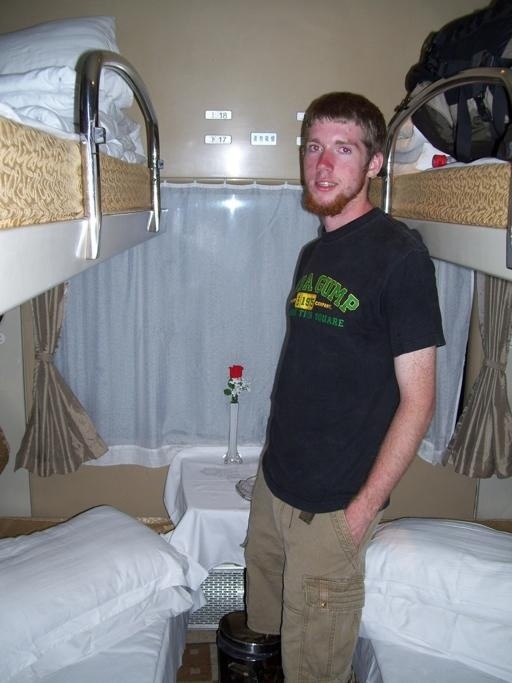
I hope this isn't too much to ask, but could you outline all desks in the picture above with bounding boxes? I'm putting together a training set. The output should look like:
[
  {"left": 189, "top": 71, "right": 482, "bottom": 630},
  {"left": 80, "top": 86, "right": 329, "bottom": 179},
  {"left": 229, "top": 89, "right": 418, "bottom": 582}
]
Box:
[{"left": 175, "top": 454, "right": 259, "bottom": 522}]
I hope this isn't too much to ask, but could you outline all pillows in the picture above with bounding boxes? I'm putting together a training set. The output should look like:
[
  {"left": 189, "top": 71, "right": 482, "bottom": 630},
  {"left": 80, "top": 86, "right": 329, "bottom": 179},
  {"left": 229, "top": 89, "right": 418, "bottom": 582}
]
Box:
[{"left": 0, "top": 504, "right": 210, "bottom": 683}]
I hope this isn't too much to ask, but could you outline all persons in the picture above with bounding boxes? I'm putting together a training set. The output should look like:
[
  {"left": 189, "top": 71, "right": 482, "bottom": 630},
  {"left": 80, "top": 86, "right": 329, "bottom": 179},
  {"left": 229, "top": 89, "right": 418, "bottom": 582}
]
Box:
[{"left": 240, "top": 91, "right": 447, "bottom": 683}]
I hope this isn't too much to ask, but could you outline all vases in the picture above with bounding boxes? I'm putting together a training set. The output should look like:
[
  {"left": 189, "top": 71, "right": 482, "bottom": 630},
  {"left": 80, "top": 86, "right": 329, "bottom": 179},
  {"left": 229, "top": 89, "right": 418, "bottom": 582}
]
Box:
[{"left": 224, "top": 402, "right": 244, "bottom": 464}]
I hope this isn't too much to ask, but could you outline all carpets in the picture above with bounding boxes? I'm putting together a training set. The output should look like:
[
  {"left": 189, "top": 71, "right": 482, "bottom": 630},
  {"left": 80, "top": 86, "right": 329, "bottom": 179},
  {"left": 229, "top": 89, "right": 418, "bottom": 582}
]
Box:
[{"left": 176, "top": 643, "right": 213, "bottom": 683}]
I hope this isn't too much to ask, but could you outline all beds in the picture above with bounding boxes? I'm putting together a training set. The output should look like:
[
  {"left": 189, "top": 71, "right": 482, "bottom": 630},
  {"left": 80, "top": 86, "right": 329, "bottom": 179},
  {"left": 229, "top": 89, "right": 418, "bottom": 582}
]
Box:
[
  {"left": 1, "top": 22, "right": 169, "bottom": 315},
  {"left": 368, "top": 65, "right": 512, "bottom": 283}
]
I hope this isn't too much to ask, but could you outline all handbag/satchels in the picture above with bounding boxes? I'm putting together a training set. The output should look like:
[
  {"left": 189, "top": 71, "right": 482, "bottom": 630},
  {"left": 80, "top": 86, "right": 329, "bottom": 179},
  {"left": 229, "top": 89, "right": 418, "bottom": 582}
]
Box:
[{"left": 405, "top": 4, "right": 511, "bottom": 162}]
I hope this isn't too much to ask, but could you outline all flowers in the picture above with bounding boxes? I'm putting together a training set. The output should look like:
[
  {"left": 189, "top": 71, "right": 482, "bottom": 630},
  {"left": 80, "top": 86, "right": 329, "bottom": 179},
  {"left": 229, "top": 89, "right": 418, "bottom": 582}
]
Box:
[{"left": 223, "top": 365, "right": 251, "bottom": 404}]
